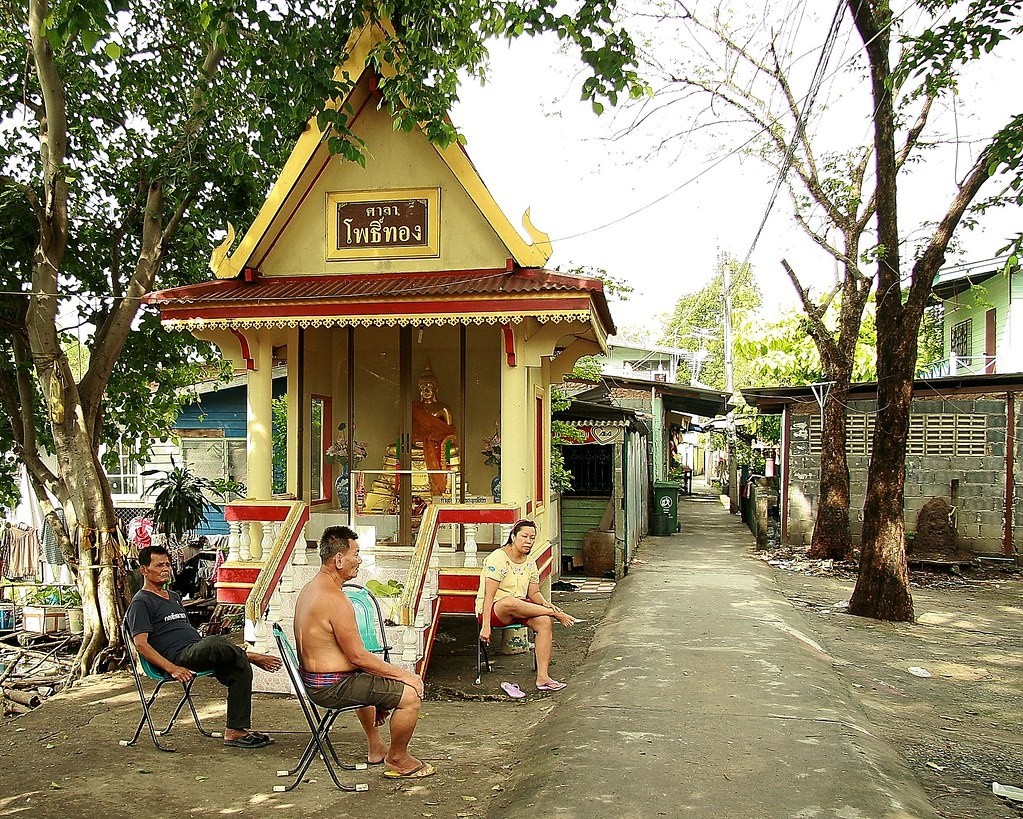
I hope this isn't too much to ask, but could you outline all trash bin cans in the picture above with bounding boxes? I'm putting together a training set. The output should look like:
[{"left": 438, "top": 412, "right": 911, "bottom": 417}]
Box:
[{"left": 653, "top": 481, "right": 681, "bottom": 533}]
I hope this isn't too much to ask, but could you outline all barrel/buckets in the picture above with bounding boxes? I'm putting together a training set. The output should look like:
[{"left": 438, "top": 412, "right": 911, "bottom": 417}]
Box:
[{"left": 584, "top": 528, "right": 615, "bottom": 575}]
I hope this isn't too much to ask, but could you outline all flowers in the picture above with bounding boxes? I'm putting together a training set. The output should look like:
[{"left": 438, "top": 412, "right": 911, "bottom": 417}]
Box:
[
  {"left": 324, "top": 421, "right": 367, "bottom": 465},
  {"left": 479, "top": 421, "right": 501, "bottom": 467}
]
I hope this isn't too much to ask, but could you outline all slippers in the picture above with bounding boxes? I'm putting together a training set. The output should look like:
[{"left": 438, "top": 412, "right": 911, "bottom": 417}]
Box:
[
  {"left": 536, "top": 678, "right": 567, "bottom": 692},
  {"left": 367, "top": 751, "right": 437, "bottom": 778},
  {"left": 500, "top": 679, "right": 525, "bottom": 698},
  {"left": 220, "top": 730, "right": 277, "bottom": 748}
]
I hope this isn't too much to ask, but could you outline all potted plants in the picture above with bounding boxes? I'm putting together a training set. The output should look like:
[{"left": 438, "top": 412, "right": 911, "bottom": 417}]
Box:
[{"left": 64, "top": 591, "right": 82, "bottom": 635}]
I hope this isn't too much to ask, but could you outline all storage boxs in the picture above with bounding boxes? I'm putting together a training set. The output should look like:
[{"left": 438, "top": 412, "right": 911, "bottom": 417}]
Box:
[{"left": 20, "top": 607, "right": 65, "bottom": 634}]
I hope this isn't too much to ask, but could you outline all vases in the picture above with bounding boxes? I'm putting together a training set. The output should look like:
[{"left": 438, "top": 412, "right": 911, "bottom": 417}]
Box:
[
  {"left": 491, "top": 468, "right": 501, "bottom": 503},
  {"left": 334, "top": 465, "right": 356, "bottom": 512}
]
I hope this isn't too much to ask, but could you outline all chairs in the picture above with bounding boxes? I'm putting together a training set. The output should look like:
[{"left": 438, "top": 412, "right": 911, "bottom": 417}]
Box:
[
  {"left": 119, "top": 617, "right": 224, "bottom": 752},
  {"left": 475, "top": 622, "right": 536, "bottom": 684},
  {"left": 272, "top": 622, "right": 369, "bottom": 792},
  {"left": 342, "top": 583, "right": 392, "bottom": 663}
]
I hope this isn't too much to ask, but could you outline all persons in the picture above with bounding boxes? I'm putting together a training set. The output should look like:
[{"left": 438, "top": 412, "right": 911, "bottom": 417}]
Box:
[
  {"left": 398, "top": 355, "right": 455, "bottom": 443},
  {"left": 127, "top": 546, "right": 283, "bottom": 748},
  {"left": 294, "top": 526, "right": 437, "bottom": 779},
  {"left": 475, "top": 520, "right": 575, "bottom": 691}
]
